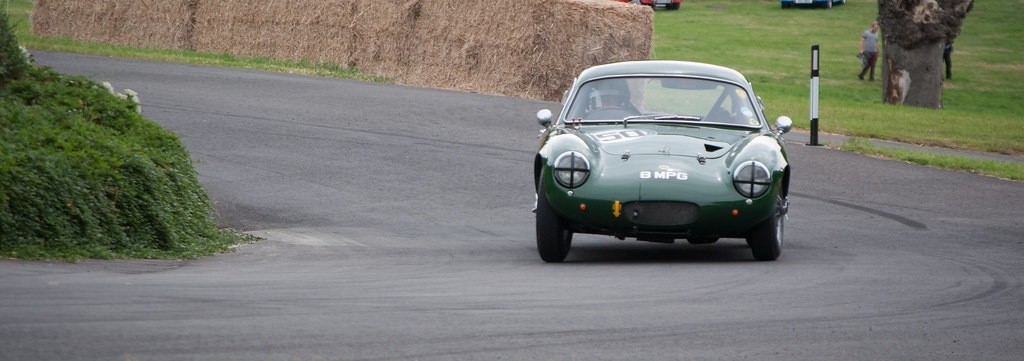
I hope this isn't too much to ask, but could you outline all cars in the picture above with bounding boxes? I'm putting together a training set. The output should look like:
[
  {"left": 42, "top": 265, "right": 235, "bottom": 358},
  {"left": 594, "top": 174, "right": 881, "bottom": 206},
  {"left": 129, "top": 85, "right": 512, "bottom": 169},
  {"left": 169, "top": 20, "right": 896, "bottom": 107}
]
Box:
[
  {"left": 530, "top": 60, "right": 792, "bottom": 261},
  {"left": 621, "top": 0, "right": 682, "bottom": 13},
  {"left": 779, "top": 1, "right": 846, "bottom": 8}
]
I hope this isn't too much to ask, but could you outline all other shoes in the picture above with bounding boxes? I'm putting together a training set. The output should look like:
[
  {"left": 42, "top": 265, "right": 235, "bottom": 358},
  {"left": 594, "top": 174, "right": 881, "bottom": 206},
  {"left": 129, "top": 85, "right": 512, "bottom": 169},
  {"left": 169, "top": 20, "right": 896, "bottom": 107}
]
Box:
[
  {"left": 869, "top": 79, "right": 875, "bottom": 81},
  {"left": 858, "top": 74, "right": 864, "bottom": 80},
  {"left": 946, "top": 78, "right": 952, "bottom": 80}
]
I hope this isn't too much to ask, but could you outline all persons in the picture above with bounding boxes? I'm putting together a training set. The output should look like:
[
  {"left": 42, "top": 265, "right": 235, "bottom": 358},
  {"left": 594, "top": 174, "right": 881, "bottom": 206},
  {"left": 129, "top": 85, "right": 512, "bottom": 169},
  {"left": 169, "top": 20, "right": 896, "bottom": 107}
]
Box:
[
  {"left": 857, "top": 22, "right": 879, "bottom": 81},
  {"left": 594, "top": 82, "right": 639, "bottom": 117},
  {"left": 944, "top": 40, "right": 954, "bottom": 79}
]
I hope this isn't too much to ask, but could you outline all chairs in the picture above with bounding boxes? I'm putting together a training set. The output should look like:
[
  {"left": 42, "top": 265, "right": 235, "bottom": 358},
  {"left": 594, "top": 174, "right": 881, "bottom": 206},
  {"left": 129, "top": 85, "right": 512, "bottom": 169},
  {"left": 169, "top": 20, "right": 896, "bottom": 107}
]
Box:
[{"left": 703, "top": 107, "right": 735, "bottom": 123}]
